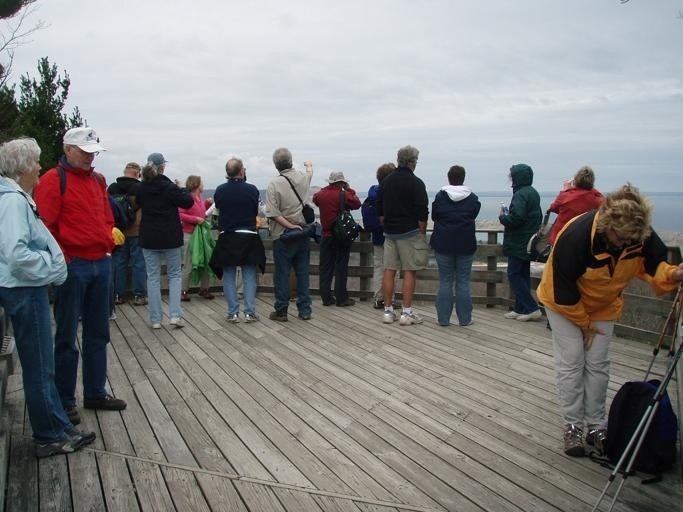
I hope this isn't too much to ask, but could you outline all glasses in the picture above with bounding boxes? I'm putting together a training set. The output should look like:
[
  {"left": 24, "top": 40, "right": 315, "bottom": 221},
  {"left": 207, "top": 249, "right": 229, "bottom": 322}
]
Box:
[{"left": 611, "top": 228, "right": 637, "bottom": 242}]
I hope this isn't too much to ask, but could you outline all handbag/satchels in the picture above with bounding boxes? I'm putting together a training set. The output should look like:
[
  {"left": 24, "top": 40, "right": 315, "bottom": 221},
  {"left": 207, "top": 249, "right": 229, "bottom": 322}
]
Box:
[
  {"left": 301, "top": 204, "right": 315, "bottom": 224},
  {"left": 526, "top": 209, "right": 552, "bottom": 263},
  {"left": 331, "top": 191, "right": 362, "bottom": 252}
]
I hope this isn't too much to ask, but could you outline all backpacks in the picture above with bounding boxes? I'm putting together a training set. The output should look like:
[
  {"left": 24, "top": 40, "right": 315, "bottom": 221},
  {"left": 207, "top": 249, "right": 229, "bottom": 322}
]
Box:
[
  {"left": 588, "top": 379, "right": 680, "bottom": 483},
  {"left": 108, "top": 183, "right": 142, "bottom": 238}
]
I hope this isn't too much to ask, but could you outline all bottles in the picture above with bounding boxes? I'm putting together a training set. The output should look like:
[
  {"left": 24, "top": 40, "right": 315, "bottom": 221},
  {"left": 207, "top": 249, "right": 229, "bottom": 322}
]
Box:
[{"left": 500, "top": 201, "right": 509, "bottom": 216}]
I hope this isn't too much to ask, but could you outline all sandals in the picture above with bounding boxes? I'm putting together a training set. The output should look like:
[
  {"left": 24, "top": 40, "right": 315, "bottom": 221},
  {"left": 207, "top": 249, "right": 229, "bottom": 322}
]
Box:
[
  {"left": 198, "top": 289, "right": 215, "bottom": 299},
  {"left": 182, "top": 292, "right": 190, "bottom": 301}
]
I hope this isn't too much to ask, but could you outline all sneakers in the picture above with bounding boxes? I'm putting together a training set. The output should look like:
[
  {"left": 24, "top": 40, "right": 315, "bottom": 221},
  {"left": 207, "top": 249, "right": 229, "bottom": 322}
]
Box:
[
  {"left": 34, "top": 394, "right": 127, "bottom": 459},
  {"left": 298, "top": 312, "right": 311, "bottom": 320},
  {"left": 504, "top": 311, "right": 518, "bottom": 319},
  {"left": 115, "top": 296, "right": 161, "bottom": 329},
  {"left": 224, "top": 310, "right": 288, "bottom": 322},
  {"left": 516, "top": 309, "right": 542, "bottom": 321},
  {"left": 373, "top": 300, "right": 423, "bottom": 325},
  {"left": 586, "top": 430, "right": 607, "bottom": 453},
  {"left": 563, "top": 424, "right": 586, "bottom": 457},
  {"left": 169, "top": 317, "right": 185, "bottom": 327},
  {"left": 336, "top": 298, "right": 355, "bottom": 307},
  {"left": 323, "top": 297, "right": 335, "bottom": 305}
]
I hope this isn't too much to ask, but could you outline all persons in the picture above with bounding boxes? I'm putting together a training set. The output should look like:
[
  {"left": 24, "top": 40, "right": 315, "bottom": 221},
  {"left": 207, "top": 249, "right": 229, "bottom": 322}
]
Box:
[
  {"left": 429, "top": 166, "right": 481, "bottom": 326},
  {"left": 547, "top": 166, "right": 607, "bottom": 330},
  {"left": 32, "top": 127, "right": 127, "bottom": 425},
  {"left": 0, "top": 136, "right": 96, "bottom": 458},
  {"left": 312, "top": 171, "right": 362, "bottom": 307},
  {"left": 536, "top": 183, "right": 683, "bottom": 457},
  {"left": 361, "top": 163, "right": 402, "bottom": 310},
  {"left": 499, "top": 164, "right": 543, "bottom": 322},
  {"left": 376, "top": 145, "right": 431, "bottom": 325},
  {"left": 99, "top": 147, "right": 314, "bottom": 330}
]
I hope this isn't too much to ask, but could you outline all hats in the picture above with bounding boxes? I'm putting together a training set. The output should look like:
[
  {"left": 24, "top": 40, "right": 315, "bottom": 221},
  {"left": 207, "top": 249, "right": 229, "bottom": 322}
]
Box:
[
  {"left": 62, "top": 127, "right": 108, "bottom": 153},
  {"left": 146, "top": 153, "right": 168, "bottom": 165},
  {"left": 125, "top": 162, "right": 141, "bottom": 171},
  {"left": 324, "top": 171, "right": 347, "bottom": 184}
]
